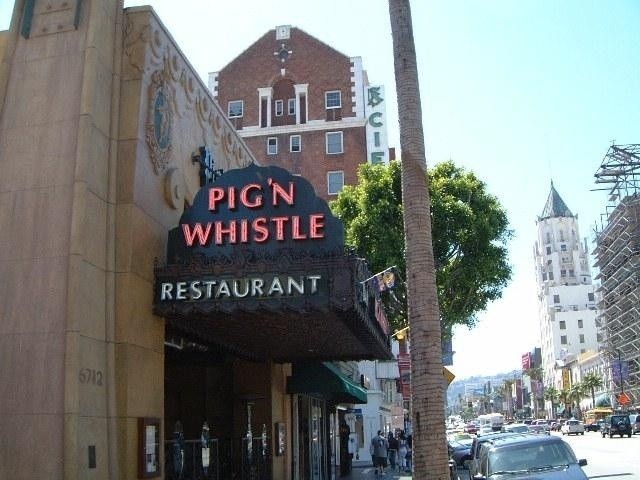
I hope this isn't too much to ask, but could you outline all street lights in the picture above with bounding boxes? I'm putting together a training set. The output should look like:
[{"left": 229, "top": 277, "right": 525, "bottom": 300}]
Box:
[{"left": 598, "top": 345, "right": 624, "bottom": 406}]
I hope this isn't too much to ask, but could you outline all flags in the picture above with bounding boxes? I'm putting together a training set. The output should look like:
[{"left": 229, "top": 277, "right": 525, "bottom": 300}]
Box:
[
  {"left": 368, "top": 267, "right": 401, "bottom": 294},
  {"left": 396, "top": 329, "right": 407, "bottom": 339}
]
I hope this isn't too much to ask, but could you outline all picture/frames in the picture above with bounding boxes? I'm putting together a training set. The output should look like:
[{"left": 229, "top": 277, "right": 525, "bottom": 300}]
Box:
[
  {"left": 137, "top": 416, "right": 162, "bottom": 479},
  {"left": 275, "top": 421, "right": 287, "bottom": 455}
]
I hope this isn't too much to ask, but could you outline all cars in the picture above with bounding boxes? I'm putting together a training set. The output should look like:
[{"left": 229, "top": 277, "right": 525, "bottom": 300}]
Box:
[
  {"left": 584, "top": 406, "right": 640, "bottom": 438},
  {"left": 443, "top": 406, "right": 589, "bottom": 480}
]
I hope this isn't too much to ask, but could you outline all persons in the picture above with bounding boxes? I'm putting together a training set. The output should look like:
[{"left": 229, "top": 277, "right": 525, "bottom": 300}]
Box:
[{"left": 370, "top": 428, "right": 411, "bottom": 475}]
